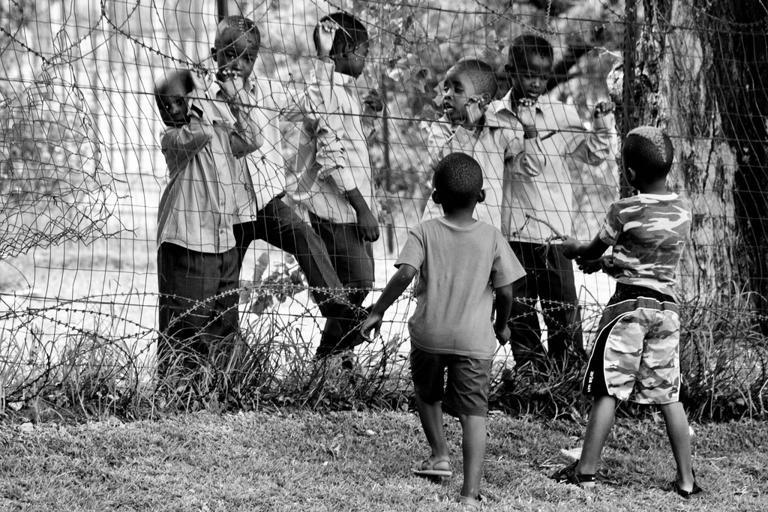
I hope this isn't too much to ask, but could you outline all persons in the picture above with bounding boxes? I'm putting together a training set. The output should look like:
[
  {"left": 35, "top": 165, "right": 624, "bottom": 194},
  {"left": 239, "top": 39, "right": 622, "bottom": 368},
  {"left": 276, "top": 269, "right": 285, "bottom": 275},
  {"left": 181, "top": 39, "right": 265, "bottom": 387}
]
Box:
[
  {"left": 360, "top": 153, "right": 527, "bottom": 504},
  {"left": 292, "top": 14, "right": 383, "bottom": 369},
  {"left": 548, "top": 126, "right": 695, "bottom": 495},
  {"left": 484, "top": 35, "right": 616, "bottom": 382},
  {"left": 202, "top": 16, "right": 350, "bottom": 317},
  {"left": 154, "top": 63, "right": 263, "bottom": 379},
  {"left": 420, "top": 56, "right": 547, "bottom": 233}
]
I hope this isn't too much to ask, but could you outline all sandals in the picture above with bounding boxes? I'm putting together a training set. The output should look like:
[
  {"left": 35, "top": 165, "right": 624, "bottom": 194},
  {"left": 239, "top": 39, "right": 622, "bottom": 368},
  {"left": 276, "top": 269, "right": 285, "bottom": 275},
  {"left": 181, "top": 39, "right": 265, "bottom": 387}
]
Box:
[
  {"left": 548, "top": 459, "right": 596, "bottom": 488},
  {"left": 673, "top": 469, "right": 702, "bottom": 498},
  {"left": 413, "top": 454, "right": 453, "bottom": 476},
  {"left": 458, "top": 492, "right": 486, "bottom": 508}
]
